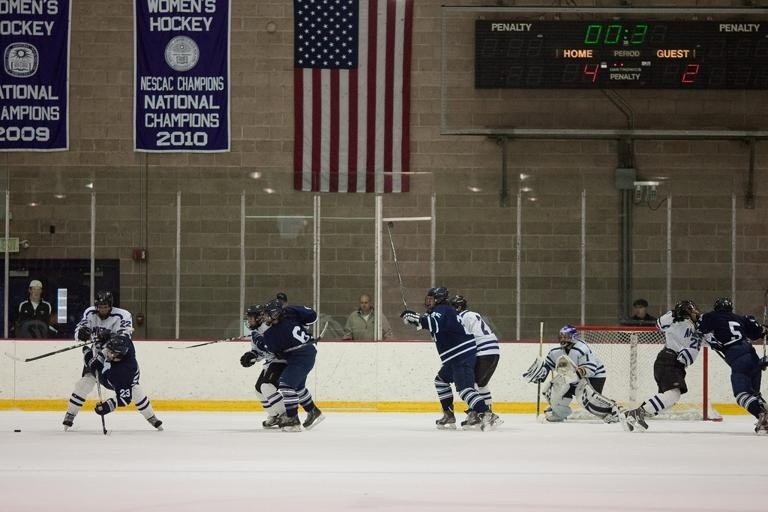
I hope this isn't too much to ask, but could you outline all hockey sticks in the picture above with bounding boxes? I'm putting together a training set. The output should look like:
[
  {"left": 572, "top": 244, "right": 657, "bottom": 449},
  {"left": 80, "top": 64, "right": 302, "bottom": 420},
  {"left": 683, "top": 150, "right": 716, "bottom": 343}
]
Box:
[
  {"left": 93, "top": 347, "right": 111, "bottom": 435},
  {"left": 4, "top": 341, "right": 94, "bottom": 363},
  {"left": 167, "top": 334, "right": 251, "bottom": 350},
  {"left": 536, "top": 321, "right": 550, "bottom": 425},
  {"left": 386, "top": 222, "right": 409, "bottom": 312}
]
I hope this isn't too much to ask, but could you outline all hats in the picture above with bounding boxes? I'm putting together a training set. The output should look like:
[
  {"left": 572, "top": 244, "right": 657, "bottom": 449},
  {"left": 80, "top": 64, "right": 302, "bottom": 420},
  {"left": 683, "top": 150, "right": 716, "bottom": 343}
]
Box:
[{"left": 29, "top": 280, "right": 42, "bottom": 288}]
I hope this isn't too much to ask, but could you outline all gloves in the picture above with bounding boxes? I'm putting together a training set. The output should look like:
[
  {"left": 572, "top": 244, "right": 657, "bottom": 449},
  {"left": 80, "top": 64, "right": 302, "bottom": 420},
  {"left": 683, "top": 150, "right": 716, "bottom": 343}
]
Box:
[
  {"left": 400, "top": 310, "right": 423, "bottom": 331},
  {"left": 96, "top": 398, "right": 116, "bottom": 414},
  {"left": 88, "top": 352, "right": 105, "bottom": 377},
  {"left": 241, "top": 352, "right": 257, "bottom": 367},
  {"left": 79, "top": 327, "right": 91, "bottom": 341},
  {"left": 673, "top": 304, "right": 689, "bottom": 322}
]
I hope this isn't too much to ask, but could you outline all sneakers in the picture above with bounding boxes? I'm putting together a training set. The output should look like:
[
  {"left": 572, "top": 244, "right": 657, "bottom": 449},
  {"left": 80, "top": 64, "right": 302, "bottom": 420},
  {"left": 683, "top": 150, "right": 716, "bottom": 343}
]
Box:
[
  {"left": 622, "top": 408, "right": 649, "bottom": 431},
  {"left": 63, "top": 412, "right": 75, "bottom": 426},
  {"left": 436, "top": 405, "right": 499, "bottom": 429},
  {"left": 262, "top": 406, "right": 320, "bottom": 428},
  {"left": 149, "top": 415, "right": 162, "bottom": 427},
  {"left": 754, "top": 408, "right": 768, "bottom": 433}
]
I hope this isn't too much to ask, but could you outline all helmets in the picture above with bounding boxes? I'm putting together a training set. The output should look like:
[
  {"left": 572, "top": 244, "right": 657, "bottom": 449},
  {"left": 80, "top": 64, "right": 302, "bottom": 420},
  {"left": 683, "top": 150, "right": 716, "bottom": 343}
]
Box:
[
  {"left": 450, "top": 294, "right": 467, "bottom": 312},
  {"left": 713, "top": 297, "right": 733, "bottom": 311},
  {"left": 424, "top": 287, "right": 448, "bottom": 308},
  {"left": 682, "top": 299, "right": 696, "bottom": 311},
  {"left": 560, "top": 325, "right": 578, "bottom": 344},
  {"left": 103, "top": 337, "right": 128, "bottom": 363},
  {"left": 95, "top": 290, "right": 113, "bottom": 320},
  {"left": 244, "top": 299, "right": 283, "bottom": 330}
]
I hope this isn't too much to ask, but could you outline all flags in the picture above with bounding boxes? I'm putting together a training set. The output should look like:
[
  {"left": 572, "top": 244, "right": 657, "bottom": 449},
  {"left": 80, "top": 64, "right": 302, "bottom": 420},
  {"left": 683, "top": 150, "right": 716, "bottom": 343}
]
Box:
[{"left": 293, "top": 0, "right": 414, "bottom": 193}]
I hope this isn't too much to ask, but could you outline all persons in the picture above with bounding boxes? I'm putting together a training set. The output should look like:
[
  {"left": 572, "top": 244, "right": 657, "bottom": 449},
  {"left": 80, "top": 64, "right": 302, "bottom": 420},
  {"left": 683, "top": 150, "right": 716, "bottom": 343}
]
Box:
[
  {"left": 251, "top": 299, "right": 321, "bottom": 428},
  {"left": 342, "top": 293, "right": 393, "bottom": 341},
  {"left": 533, "top": 324, "right": 621, "bottom": 422},
  {"left": 402, "top": 285, "right": 498, "bottom": 430},
  {"left": 63, "top": 291, "right": 162, "bottom": 428},
  {"left": 692, "top": 296, "right": 768, "bottom": 432},
  {"left": 276, "top": 293, "right": 288, "bottom": 308},
  {"left": 624, "top": 299, "right": 701, "bottom": 432},
  {"left": 452, "top": 294, "right": 500, "bottom": 426},
  {"left": 621, "top": 299, "right": 659, "bottom": 343},
  {"left": 241, "top": 303, "right": 287, "bottom": 427},
  {"left": 83, "top": 330, "right": 139, "bottom": 413},
  {"left": 19, "top": 281, "right": 51, "bottom": 339}
]
